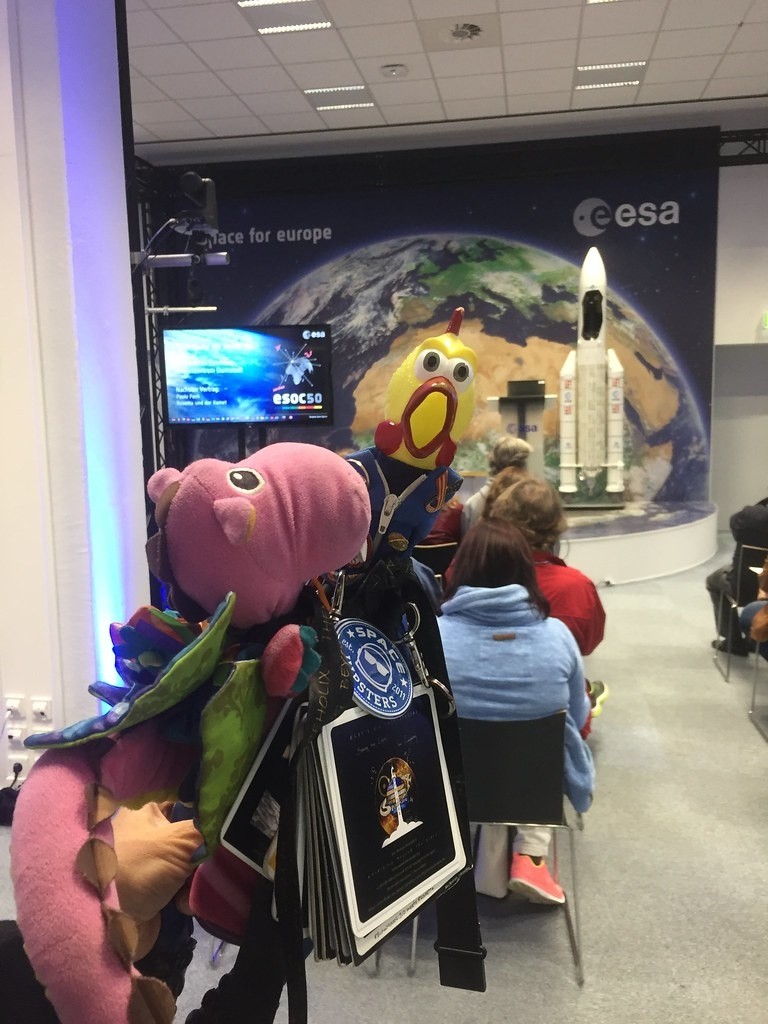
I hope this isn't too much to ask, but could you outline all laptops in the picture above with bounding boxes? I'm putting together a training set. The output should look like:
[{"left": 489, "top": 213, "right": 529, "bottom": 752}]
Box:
[{"left": 508, "top": 380, "right": 545, "bottom": 396}]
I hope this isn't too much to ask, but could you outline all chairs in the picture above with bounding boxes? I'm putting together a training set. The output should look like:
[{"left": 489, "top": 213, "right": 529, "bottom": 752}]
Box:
[
  {"left": 748, "top": 642, "right": 761, "bottom": 713},
  {"left": 412, "top": 542, "right": 459, "bottom": 592},
  {"left": 714, "top": 545, "right": 768, "bottom": 681},
  {"left": 409, "top": 709, "right": 580, "bottom": 973}
]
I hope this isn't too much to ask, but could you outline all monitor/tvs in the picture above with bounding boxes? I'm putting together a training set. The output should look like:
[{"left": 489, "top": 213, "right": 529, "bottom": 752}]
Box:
[{"left": 159, "top": 324, "right": 334, "bottom": 428}]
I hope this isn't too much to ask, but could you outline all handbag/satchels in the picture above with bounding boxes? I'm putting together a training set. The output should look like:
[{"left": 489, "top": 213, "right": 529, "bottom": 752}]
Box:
[{"left": 474, "top": 824, "right": 517, "bottom": 899}]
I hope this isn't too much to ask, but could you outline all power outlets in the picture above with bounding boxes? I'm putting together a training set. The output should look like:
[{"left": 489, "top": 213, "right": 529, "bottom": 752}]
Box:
[
  {"left": 7, "top": 755, "right": 29, "bottom": 777},
  {"left": 6, "top": 694, "right": 26, "bottom": 720},
  {"left": 33, "top": 728, "right": 54, "bottom": 750},
  {"left": 31, "top": 697, "right": 52, "bottom": 722},
  {"left": 7, "top": 724, "right": 29, "bottom": 750},
  {"left": 6, "top": 778, "right": 28, "bottom": 793}
]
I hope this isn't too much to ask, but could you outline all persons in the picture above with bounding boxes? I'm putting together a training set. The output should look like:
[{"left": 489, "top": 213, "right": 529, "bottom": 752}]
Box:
[
  {"left": 445, "top": 435, "right": 606, "bottom": 656},
  {"left": 433, "top": 516, "right": 589, "bottom": 906},
  {"left": 706, "top": 498, "right": 767, "bottom": 658},
  {"left": 420, "top": 497, "right": 463, "bottom": 546}
]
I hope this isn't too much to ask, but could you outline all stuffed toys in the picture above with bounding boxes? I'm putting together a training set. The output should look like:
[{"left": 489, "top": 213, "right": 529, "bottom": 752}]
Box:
[{"left": 0, "top": 441, "right": 370, "bottom": 1024}]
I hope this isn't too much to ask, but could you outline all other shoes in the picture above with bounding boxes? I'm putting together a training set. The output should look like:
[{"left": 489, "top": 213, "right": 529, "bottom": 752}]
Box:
[
  {"left": 711, "top": 639, "right": 749, "bottom": 656},
  {"left": 507, "top": 853, "right": 565, "bottom": 906},
  {"left": 585, "top": 681, "right": 608, "bottom": 718}
]
